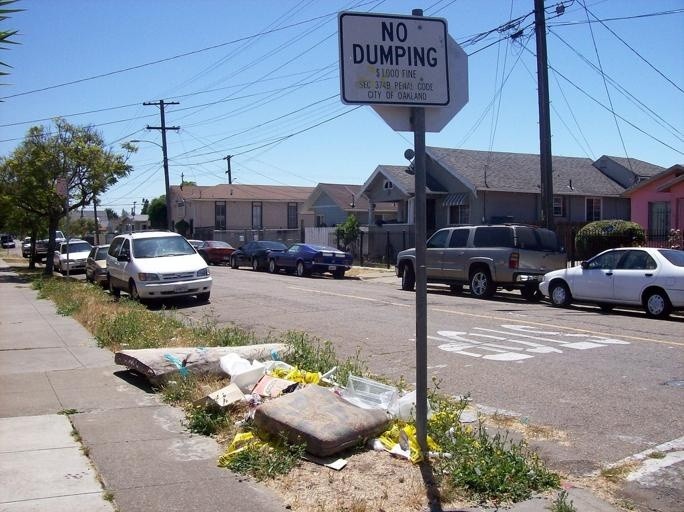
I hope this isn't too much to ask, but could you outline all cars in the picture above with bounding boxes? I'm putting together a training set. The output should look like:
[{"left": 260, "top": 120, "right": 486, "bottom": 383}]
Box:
[
  {"left": 1, "top": 233, "right": 15, "bottom": 248},
  {"left": 22, "top": 230, "right": 213, "bottom": 305},
  {"left": 186, "top": 238, "right": 353, "bottom": 280},
  {"left": 539, "top": 245, "right": 684, "bottom": 317}
]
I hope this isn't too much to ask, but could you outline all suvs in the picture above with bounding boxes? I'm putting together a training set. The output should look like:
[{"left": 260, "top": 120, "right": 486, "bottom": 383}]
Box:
[{"left": 395, "top": 221, "right": 565, "bottom": 301}]
